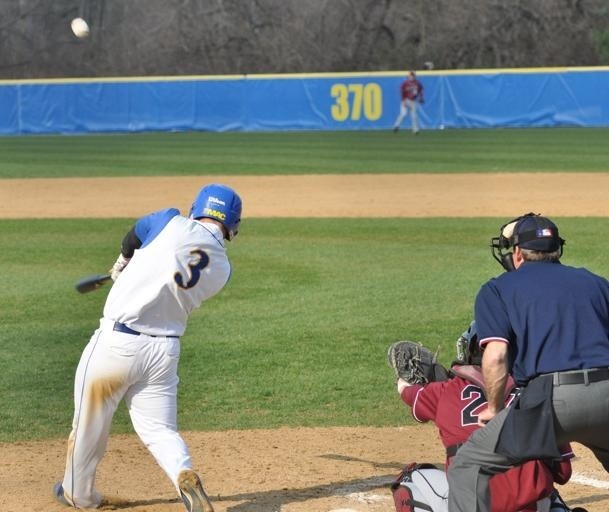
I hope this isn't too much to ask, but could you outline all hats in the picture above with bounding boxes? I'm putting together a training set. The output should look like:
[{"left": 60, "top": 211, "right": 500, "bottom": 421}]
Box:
[{"left": 514, "top": 216, "right": 562, "bottom": 251}]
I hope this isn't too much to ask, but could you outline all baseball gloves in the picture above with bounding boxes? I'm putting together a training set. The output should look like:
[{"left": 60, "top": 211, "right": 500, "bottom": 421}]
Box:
[{"left": 388, "top": 341, "right": 448, "bottom": 386}]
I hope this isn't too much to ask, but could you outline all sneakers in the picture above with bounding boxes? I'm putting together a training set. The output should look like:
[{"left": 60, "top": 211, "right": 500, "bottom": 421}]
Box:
[
  {"left": 178, "top": 471, "right": 214, "bottom": 512},
  {"left": 52, "top": 482, "right": 72, "bottom": 506}
]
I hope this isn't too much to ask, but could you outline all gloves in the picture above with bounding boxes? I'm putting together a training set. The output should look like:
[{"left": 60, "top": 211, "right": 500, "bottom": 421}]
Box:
[{"left": 111, "top": 253, "right": 129, "bottom": 282}]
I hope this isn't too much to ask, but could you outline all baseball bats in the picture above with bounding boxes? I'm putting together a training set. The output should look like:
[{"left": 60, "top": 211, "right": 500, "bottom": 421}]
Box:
[{"left": 76, "top": 275, "right": 110, "bottom": 293}]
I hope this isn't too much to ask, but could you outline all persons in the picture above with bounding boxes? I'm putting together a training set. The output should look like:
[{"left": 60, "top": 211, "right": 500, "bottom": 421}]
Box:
[
  {"left": 447, "top": 212, "right": 609, "bottom": 511},
  {"left": 51, "top": 184, "right": 242, "bottom": 511},
  {"left": 391, "top": 321, "right": 567, "bottom": 512},
  {"left": 392, "top": 71, "right": 423, "bottom": 134}
]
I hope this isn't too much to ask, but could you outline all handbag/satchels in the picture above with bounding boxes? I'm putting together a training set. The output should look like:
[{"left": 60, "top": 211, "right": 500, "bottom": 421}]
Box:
[{"left": 494, "top": 398, "right": 562, "bottom": 467}]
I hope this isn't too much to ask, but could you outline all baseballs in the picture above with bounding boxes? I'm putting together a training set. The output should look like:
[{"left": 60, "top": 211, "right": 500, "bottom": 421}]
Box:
[{"left": 71, "top": 18, "right": 89, "bottom": 37}]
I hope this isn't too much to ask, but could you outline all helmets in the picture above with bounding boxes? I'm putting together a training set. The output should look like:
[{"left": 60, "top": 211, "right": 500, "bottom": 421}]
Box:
[
  {"left": 189, "top": 184, "right": 242, "bottom": 241},
  {"left": 456, "top": 321, "right": 480, "bottom": 364}
]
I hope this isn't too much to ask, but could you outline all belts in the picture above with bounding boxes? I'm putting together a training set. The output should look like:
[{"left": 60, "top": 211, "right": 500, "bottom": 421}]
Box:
[
  {"left": 113, "top": 321, "right": 179, "bottom": 337},
  {"left": 558, "top": 369, "right": 609, "bottom": 385}
]
